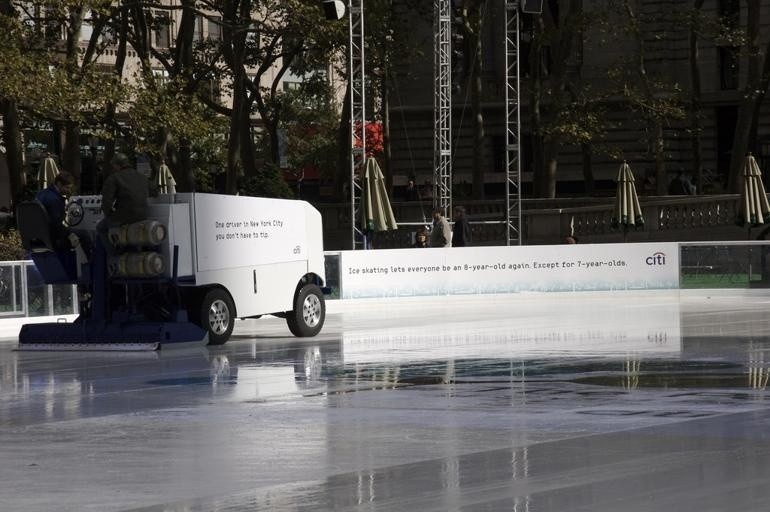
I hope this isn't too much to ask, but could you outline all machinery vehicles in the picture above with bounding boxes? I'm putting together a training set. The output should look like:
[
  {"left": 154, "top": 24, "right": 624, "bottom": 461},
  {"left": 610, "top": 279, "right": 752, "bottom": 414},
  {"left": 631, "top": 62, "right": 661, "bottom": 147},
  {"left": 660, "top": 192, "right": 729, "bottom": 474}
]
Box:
[{"left": 12, "top": 171, "right": 334, "bottom": 356}]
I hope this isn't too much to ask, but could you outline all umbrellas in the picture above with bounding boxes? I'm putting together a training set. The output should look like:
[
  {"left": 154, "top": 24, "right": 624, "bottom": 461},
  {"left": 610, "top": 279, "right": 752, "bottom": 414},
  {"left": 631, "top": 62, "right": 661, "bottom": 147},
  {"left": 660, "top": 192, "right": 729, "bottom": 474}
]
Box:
[
  {"left": 154, "top": 160, "right": 176, "bottom": 193},
  {"left": 36, "top": 151, "right": 70, "bottom": 234},
  {"left": 735, "top": 151, "right": 770, "bottom": 285},
  {"left": 609, "top": 157, "right": 646, "bottom": 242},
  {"left": 358, "top": 155, "right": 399, "bottom": 249}
]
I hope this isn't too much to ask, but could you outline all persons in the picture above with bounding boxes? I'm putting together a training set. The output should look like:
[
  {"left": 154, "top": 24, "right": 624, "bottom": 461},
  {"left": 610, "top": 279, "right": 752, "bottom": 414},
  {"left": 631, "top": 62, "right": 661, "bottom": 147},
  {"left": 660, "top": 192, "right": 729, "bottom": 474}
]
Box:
[
  {"left": 37, "top": 173, "right": 75, "bottom": 242},
  {"left": 96, "top": 151, "right": 151, "bottom": 256},
  {"left": 451, "top": 205, "right": 473, "bottom": 247},
  {"left": 429, "top": 206, "right": 452, "bottom": 248},
  {"left": 411, "top": 230, "right": 430, "bottom": 247}
]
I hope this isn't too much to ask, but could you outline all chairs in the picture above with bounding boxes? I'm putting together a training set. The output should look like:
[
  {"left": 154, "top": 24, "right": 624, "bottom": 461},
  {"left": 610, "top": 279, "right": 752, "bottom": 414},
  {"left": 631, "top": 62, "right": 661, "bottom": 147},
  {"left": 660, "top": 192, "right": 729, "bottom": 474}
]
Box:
[{"left": 16, "top": 200, "right": 92, "bottom": 284}]
